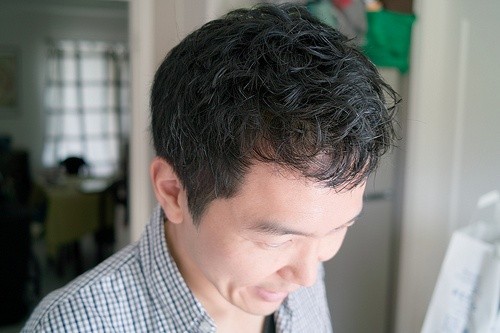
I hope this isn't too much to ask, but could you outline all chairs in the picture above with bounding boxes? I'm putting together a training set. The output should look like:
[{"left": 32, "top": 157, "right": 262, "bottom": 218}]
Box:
[{"left": 73, "top": 144, "right": 129, "bottom": 267}]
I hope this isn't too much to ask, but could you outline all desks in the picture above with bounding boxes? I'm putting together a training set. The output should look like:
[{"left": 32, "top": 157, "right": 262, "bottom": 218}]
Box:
[{"left": 34, "top": 176, "right": 115, "bottom": 260}]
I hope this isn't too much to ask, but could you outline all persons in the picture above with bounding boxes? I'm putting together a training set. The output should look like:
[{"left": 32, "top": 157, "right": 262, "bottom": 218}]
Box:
[{"left": 21, "top": 4, "right": 401, "bottom": 333}]
